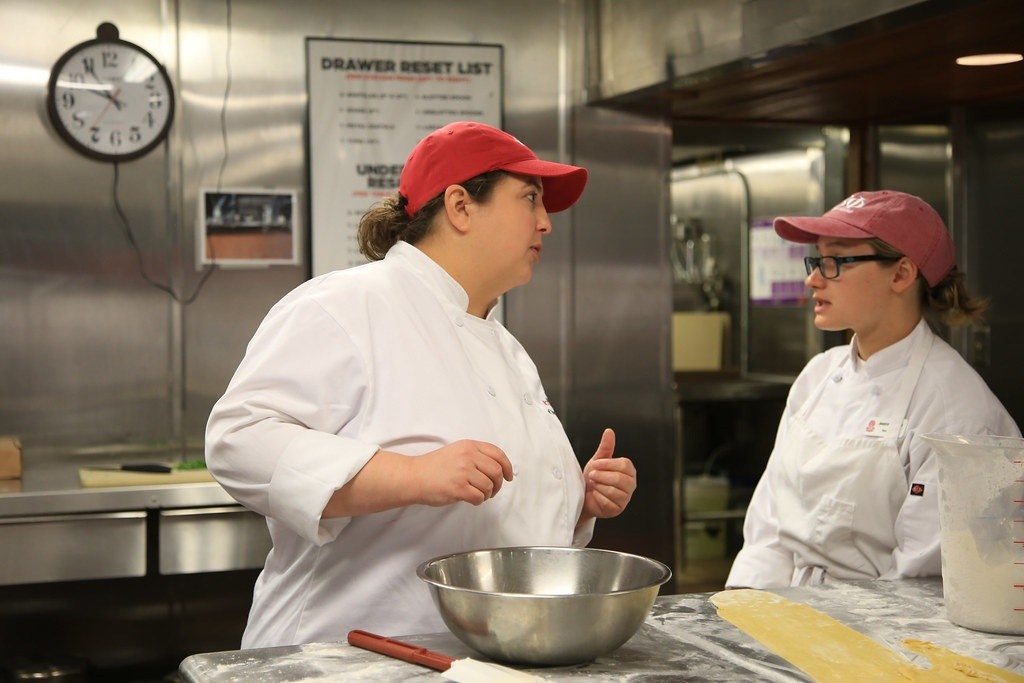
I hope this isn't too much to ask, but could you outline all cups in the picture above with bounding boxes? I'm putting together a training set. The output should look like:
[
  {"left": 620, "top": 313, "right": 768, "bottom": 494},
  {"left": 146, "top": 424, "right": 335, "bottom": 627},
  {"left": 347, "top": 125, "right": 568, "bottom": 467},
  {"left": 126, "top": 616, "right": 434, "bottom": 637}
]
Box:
[{"left": 919, "top": 431, "right": 1024, "bottom": 636}]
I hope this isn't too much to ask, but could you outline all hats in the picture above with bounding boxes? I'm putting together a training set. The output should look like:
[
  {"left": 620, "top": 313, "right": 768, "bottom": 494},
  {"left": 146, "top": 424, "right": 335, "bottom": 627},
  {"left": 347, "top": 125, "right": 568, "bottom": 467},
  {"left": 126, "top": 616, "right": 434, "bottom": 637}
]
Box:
[
  {"left": 775, "top": 191, "right": 957, "bottom": 289},
  {"left": 397, "top": 122, "right": 588, "bottom": 217}
]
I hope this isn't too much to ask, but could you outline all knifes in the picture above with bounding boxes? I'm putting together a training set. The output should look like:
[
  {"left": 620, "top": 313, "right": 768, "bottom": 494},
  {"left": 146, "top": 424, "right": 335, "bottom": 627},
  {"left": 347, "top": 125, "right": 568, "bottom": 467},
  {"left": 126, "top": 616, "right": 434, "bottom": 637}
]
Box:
[{"left": 80, "top": 460, "right": 174, "bottom": 473}]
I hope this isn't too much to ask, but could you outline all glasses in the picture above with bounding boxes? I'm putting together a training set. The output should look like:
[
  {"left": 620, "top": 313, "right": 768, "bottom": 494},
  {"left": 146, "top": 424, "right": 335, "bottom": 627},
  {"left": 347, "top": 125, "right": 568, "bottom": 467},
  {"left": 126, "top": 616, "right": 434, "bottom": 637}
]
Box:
[{"left": 803, "top": 255, "right": 894, "bottom": 278}]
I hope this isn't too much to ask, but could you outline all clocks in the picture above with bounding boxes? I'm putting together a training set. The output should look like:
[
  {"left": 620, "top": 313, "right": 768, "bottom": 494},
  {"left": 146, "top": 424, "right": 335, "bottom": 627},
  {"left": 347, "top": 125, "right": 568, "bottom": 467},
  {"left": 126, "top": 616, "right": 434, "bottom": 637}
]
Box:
[{"left": 48, "top": 38, "right": 175, "bottom": 162}]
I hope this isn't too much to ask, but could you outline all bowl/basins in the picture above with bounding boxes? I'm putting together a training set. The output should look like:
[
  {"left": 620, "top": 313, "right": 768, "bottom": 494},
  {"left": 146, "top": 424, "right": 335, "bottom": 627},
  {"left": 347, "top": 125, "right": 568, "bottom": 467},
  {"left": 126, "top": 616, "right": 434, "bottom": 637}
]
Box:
[{"left": 416, "top": 545, "right": 673, "bottom": 669}]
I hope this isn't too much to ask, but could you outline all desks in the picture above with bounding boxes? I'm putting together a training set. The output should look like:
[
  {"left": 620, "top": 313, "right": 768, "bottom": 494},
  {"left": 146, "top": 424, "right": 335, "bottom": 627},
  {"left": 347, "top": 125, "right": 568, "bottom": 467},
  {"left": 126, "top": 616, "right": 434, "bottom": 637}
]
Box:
[{"left": 179, "top": 576, "right": 1022, "bottom": 683}]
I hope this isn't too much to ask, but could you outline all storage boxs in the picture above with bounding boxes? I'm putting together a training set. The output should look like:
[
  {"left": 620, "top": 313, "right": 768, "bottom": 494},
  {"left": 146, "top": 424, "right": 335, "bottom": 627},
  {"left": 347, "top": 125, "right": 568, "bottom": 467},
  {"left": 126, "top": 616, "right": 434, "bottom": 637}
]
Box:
[
  {"left": 682, "top": 520, "right": 727, "bottom": 559},
  {"left": 674, "top": 310, "right": 729, "bottom": 373},
  {"left": 681, "top": 474, "right": 729, "bottom": 511}
]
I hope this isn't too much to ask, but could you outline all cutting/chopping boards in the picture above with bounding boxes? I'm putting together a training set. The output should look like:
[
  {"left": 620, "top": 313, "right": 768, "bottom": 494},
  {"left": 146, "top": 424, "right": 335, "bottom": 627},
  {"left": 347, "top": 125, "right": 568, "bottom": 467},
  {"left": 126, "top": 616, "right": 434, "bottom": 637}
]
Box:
[{"left": 77, "top": 459, "right": 218, "bottom": 488}]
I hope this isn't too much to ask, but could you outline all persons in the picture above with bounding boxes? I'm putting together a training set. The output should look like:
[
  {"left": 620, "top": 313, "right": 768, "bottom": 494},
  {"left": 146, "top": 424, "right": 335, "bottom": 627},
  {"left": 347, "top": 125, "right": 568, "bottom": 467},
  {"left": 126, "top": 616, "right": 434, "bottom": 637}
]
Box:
[
  {"left": 724, "top": 189, "right": 1024, "bottom": 591},
  {"left": 205, "top": 121, "right": 636, "bottom": 651}
]
